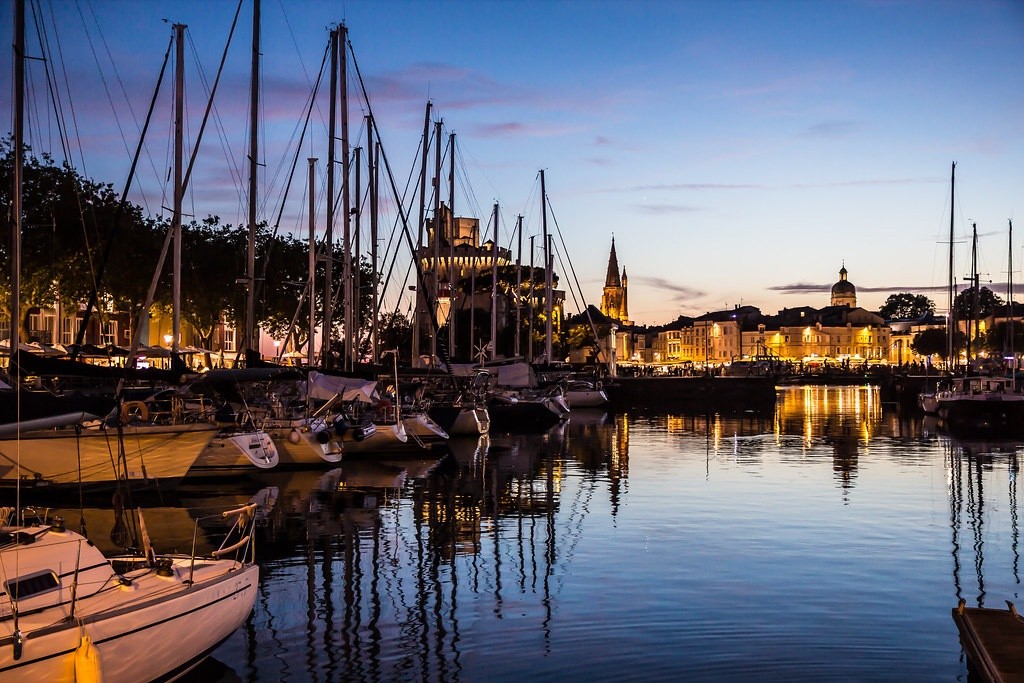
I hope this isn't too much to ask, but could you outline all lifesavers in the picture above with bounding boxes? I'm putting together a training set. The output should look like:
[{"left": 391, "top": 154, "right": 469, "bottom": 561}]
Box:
[
  {"left": 376, "top": 397, "right": 393, "bottom": 415},
  {"left": 595, "top": 381, "right": 603, "bottom": 390},
  {"left": 121, "top": 400, "right": 149, "bottom": 424}
]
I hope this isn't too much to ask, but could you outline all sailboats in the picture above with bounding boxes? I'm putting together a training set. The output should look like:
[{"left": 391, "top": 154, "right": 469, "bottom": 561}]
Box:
[
  {"left": 917, "top": 159, "right": 1024, "bottom": 426},
  {"left": 0, "top": 0, "right": 615, "bottom": 682}
]
